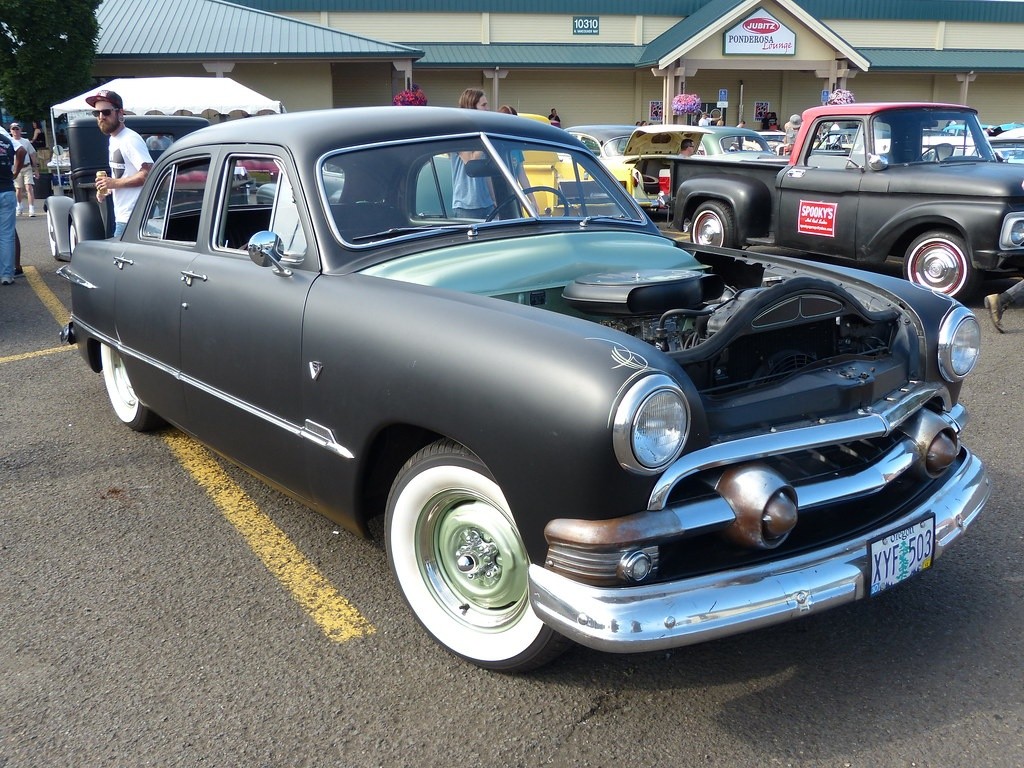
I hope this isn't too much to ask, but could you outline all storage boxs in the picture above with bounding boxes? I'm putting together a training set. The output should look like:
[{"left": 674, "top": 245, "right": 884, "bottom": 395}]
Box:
[{"left": 52, "top": 174, "right": 72, "bottom": 186}]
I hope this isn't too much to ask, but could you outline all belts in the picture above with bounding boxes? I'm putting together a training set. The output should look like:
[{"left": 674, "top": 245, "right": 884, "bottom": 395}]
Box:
[{"left": 21, "top": 163, "right": 32, "bottom": 168}]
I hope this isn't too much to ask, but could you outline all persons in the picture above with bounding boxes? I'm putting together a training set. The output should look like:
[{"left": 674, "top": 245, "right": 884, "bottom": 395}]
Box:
[
  {"left": 85, "top": 90, "right": 154, "bottom": 237},
  {"left": 146, "top": 135, "right": 173, "bottom": 149},
  {"left": 29, "top": 120, "right": 46, "bottom": 149},
  {"left": 735, "top": 121, "right": 745, "bottom": 141},
  {"left": 679, "top": 139, "right": 694, "bottom": 157},
  {"left": 985, "top": 279, "right": 1024, "bottom": 333},
  {"left": 636, "top": 121, "right": 653, "bottom": 126},
  {"left": 451, "top": 88, "right": 500, "bottom": 219},
  {"left": 829, "top": 122, "right": 841, "bottom": 143},
  {"left": 699, "top": 112, "right": 724, "bottom": 126},
  {"left": 984, "top": 126, "right": 994, "bottom": 136},
  {"left": 10, "top": 123, "right": 40, "bottom": 217},
  {"left": 491, "top": 105, "right": 538, "bottom": 218},
  {"left": 0, "top": 125, "right": 27, "bottom": 284},
  {"left": 549, "top": 109, "right": 560, "bottom": 121},
  {"left": 761, "top": 112, "right": 777, "bottom": 130},
  {"left": 784, "top": 114, "right": 801, "bottom": 152}
]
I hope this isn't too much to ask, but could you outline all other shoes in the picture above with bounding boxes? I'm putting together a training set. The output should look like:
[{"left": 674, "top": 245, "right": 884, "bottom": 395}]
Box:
[
  {"left": 14, "top": 265, "right": 24, "bottom": 277},
  {"left": 28, "top": 210, "right": 37, "bottom": 218},
  {"left": 15, "top": 206, "right": 23, "bottom": 216},
  {"left": 984, "top": 294, "right": 1006, "bottom": 334}
]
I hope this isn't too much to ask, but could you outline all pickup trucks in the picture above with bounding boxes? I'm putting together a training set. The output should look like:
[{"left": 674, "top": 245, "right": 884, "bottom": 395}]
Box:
[{"left": 663, "top": 97, "right": 1024, "bottom": 302}]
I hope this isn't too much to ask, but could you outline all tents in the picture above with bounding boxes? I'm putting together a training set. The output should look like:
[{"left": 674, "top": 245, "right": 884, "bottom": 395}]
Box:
[{"left": 49, "top": 78, "right": 290, "bottom": 187}]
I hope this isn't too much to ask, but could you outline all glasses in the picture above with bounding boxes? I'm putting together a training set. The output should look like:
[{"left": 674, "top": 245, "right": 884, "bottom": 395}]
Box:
[{"left": 92, "top": 109, "right": 120, "bottom": 117}]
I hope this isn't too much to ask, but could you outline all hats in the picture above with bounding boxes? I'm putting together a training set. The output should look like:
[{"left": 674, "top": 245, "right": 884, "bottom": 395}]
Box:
[
  {"left": 85, "top": 90, "right": 123, "bottom": 110},
  {"left": 787, "top": 114, "right": 802, "bottom": 129},
  {"left": 9, "top": 123, "right": 21, "bottom": 130}
]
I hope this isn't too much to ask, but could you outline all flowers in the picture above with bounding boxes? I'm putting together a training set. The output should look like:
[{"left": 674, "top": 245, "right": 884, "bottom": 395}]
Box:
[
  {"left": 671, "top": 94, "right": 702, "bottom": 116},
  {"left": 391, "top": 83, "right": 428, "bottom": 106},
  {"left": 826, "top": 89, "right": 856, "bottom": 105}
]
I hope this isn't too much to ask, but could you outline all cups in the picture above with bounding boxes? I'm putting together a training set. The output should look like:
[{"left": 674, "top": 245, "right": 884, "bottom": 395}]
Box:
[{"left": 96, "top": 171, "right": 108, "bottom": 195}]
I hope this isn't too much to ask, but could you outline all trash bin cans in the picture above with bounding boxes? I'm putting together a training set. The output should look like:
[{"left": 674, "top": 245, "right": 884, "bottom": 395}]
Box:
[{"left": 30, "top": 173, "right": 54, "bottom": 200}]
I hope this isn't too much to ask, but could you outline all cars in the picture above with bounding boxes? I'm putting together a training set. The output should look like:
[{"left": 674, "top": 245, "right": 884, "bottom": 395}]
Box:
[
  {"left": 232, "top": 111, "right": 1024, "bottom": 218},
  {"left": 47, "top": 116, "right": 256, "bottom": 265},
  {"left": 56, "top": 105, "right": 992, "bottom": 678}
]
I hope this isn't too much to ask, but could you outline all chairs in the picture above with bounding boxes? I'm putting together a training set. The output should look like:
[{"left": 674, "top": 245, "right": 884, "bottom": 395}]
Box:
[{"left": 345, "top": 165, "right": 410, "bottom": 237}]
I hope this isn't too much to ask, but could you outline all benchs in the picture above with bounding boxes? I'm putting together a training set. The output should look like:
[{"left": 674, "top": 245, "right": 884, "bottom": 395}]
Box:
[{"left": 164, "top": 204, "right": 344, "bottom": 250}]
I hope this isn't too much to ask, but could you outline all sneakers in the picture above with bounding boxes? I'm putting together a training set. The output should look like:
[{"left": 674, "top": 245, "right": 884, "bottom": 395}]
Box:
[{"left": 1, "top": 277, "right": 15, "bottom": 285}]
